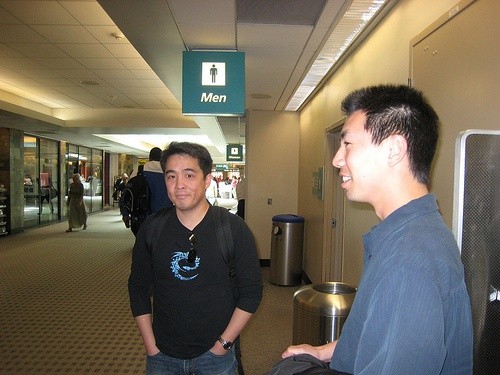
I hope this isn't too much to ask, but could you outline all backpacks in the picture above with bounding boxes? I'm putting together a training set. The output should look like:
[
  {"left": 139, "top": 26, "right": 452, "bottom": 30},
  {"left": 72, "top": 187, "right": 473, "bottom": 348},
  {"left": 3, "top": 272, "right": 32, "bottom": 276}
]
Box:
[{"left": 120, "top": 165, "right": 151, "bottom": 218}]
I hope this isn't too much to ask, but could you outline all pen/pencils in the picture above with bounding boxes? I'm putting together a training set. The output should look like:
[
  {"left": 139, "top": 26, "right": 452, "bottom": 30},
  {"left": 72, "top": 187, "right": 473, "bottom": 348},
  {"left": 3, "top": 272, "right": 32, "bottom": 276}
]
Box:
[{"left": 355, "top": 288, "right": 358, "bottom": 292}]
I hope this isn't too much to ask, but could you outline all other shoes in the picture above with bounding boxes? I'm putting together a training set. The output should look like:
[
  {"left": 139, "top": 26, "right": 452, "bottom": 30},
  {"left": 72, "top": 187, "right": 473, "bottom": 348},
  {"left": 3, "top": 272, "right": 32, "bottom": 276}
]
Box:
[
  {"left": 66, "top": 229, "right": 72, "bottom": 232},
  {"left": 83, "top": 226, "right": 86, "bottom": 230}
]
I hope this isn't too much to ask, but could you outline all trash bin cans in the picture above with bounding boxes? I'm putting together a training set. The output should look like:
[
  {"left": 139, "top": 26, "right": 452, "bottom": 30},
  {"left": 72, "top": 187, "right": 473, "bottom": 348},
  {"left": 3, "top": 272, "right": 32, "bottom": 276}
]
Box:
[
  {"left": 293, "top": 282, "right": 356, "bottom": 346},
  {"left": 269, "top": 214, "right": 305, "bottom": 286}
]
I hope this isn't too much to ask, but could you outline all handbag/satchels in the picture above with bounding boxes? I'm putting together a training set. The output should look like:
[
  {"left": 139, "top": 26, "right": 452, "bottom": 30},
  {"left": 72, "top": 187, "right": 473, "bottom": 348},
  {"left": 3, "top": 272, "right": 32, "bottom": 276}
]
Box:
[{"left": 51, "top": 186, "right": 58, "bottom": 199}]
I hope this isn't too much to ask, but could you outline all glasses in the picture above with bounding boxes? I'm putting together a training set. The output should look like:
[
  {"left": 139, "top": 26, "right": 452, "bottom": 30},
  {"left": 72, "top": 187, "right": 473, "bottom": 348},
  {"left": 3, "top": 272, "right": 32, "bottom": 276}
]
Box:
[{"left": 187, "top": 232, "right": 198, "bottom": 263}]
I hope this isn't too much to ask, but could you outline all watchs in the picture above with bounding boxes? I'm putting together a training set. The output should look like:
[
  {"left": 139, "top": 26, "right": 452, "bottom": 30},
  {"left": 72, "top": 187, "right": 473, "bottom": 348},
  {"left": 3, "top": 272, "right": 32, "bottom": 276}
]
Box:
[{"left": 217, "top": 336, "right": 233, "bottom": 350}]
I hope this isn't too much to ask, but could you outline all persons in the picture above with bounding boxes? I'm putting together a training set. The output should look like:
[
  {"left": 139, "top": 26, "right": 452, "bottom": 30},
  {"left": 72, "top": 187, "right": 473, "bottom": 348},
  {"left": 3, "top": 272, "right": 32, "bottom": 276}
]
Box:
[
  {"left": 115, "top": 173, "right": 129, "bottom": 215},
  {"left": 282, "top": 83, "right": 473, "bottom": 375},
  {"left": 231, "top": 176, "right": 238, "bottom": 198},
  {"left": 213, "top": 175, "right": 222, "bottom": 197},
  {"left": 131, "top": 147, "right": 173, "bottom": 237},
  {"left": 65, "top": 173, "right": 87, "bottom": 232},
  {"left": 128, "top": 141, "right": 264, "bottom": 375},
  {"left": 37, "top": 177, "right": 54, "bottom": 215}
]
